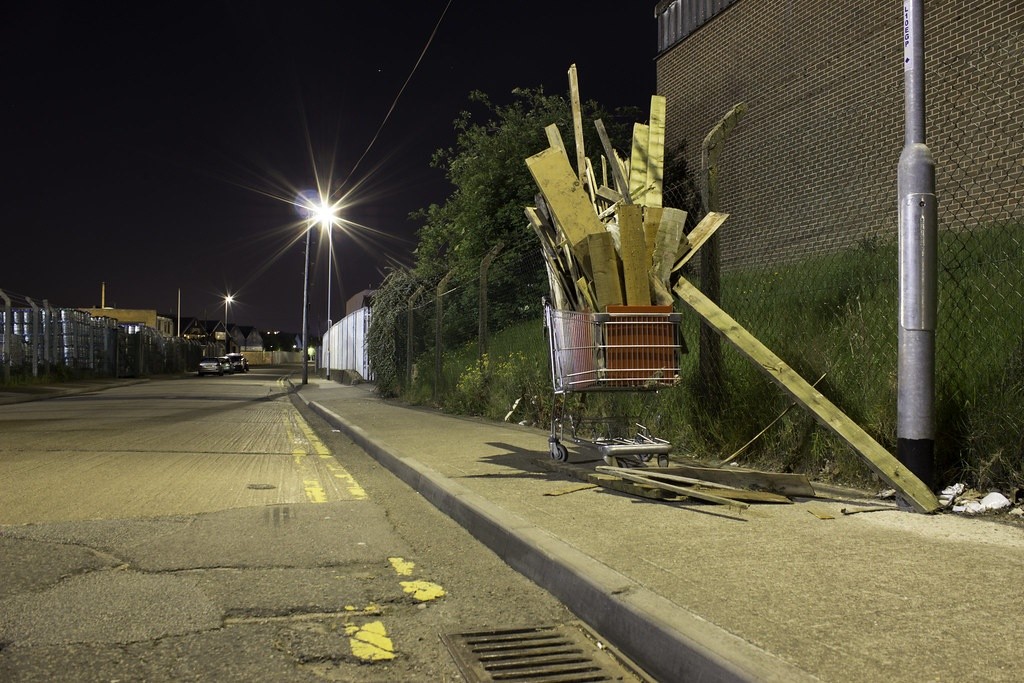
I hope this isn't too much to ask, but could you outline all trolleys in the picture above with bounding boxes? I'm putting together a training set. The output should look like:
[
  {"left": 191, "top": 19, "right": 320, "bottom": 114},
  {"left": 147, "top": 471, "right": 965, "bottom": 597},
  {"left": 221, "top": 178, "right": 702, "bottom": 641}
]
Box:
[{"left": 541, "top": 295, "right": 683, "bottom": 467}]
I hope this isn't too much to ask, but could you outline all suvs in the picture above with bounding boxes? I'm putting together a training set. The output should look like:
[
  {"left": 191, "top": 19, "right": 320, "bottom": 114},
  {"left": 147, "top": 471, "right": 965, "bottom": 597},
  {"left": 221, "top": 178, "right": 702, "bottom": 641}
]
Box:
[
  {"left": 226, "top": 354, "right": 245, "bottom": 373},
  {"left": 243, "top": 358, "right": 249, "bottom": 371}
]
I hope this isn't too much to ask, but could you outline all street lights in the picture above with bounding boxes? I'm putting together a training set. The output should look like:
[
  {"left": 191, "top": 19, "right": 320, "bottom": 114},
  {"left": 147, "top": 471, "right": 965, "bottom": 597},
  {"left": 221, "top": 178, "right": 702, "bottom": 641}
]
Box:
[
  {"left": 225, "top": 296, "right": 232, "bottom": 355},
  {"left": 303, "top": 202, "right": 335, "bottom": 384}
]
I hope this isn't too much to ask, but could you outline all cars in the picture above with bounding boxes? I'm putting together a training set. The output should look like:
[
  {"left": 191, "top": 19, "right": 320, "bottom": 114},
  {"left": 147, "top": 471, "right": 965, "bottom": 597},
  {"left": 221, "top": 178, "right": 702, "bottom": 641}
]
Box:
[
  {"left": 198, "top": 357, "right": 223, "bottom": 376},
  {"left": 218, "top": 357, "right": 235, "bottom": 374}
]
